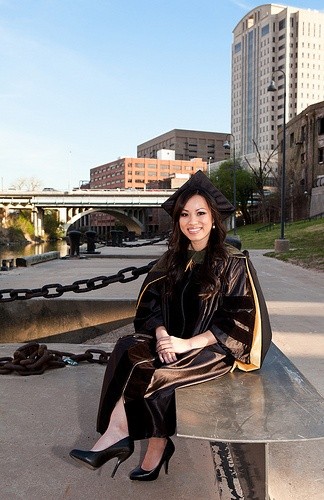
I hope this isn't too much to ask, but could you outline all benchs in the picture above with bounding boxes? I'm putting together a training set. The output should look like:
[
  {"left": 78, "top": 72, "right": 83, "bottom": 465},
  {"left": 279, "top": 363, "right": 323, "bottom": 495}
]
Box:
[
  {"left": 1, "top": 258, "right": 14, "bottom": 270},
  {"left": 168, "top": 335, "right": 324, "bottom": 500}
]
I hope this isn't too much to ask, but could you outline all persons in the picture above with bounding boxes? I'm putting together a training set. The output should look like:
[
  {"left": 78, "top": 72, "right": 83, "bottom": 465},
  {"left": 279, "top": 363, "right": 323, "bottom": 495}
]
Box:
[{"left": 69, "top": 170, "right": 272, "bottom": 482}]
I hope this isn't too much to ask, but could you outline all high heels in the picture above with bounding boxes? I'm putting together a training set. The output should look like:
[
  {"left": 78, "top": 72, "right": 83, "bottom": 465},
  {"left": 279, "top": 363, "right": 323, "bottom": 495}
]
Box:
[
  {"left": 129, "top": 437, "right": 175, "bottom": 481},
  {"left": 70, "top": 434, "right": 135, "bottom": 479}
]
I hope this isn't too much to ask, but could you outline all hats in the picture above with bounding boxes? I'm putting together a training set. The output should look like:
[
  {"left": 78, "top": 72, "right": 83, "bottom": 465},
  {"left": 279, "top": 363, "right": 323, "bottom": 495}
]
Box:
[{"left": 161, "top": 169, "right": 237, "bottom": 222}]
